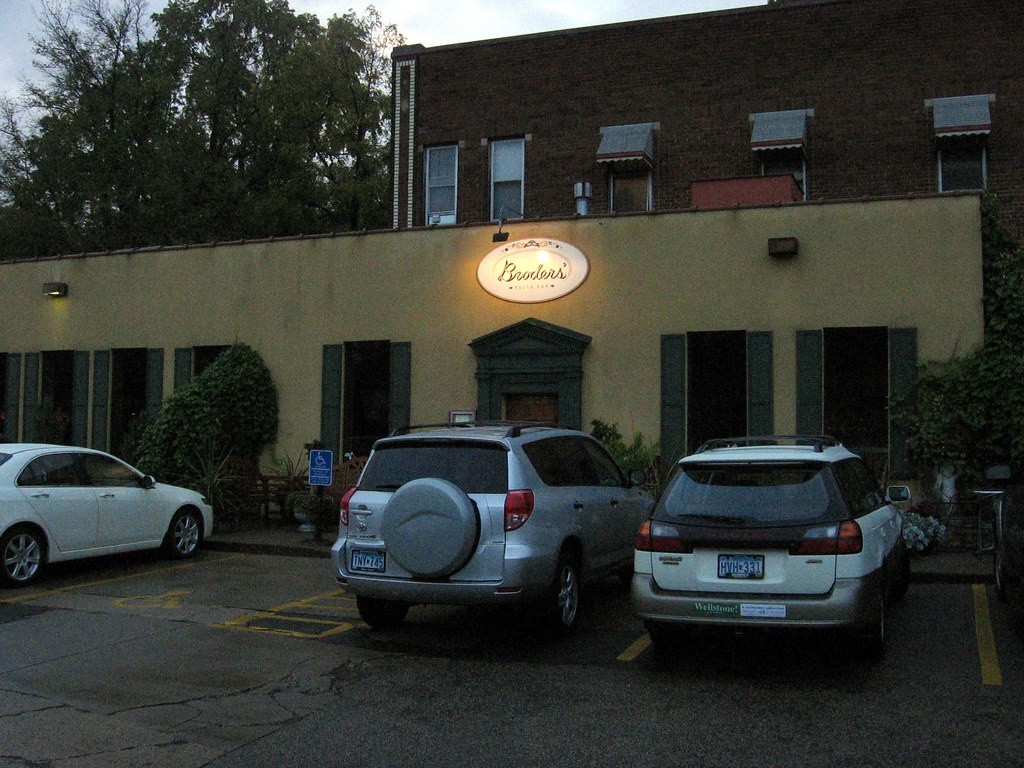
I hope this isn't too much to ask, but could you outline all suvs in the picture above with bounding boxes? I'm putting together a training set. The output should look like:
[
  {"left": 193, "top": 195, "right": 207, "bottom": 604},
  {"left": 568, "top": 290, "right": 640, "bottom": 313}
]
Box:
[
  {"left": 331, "top": 419, "right": 656, "bottom": 645},
  {"left": 628, "top": 434, "right": 911, "bottom": 662}
]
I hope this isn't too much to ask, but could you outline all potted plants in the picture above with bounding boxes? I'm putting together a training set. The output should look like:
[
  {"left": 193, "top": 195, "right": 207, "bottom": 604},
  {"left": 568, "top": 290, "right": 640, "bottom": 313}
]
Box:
[
  {"left": 164, "top": 440, "right": 238, "bottom": 532},
  {"left": 285, "top": 487, "right": 319, "bottom": 533}
]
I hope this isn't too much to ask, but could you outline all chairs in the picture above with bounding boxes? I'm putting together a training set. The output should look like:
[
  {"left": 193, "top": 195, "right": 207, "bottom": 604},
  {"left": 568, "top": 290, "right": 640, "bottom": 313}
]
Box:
[
  {"left": 734, "top": 479, "right": 764, "bottom": 507},
  {"left": 534, "top": 455, "right": 565, "bottom": 487}
]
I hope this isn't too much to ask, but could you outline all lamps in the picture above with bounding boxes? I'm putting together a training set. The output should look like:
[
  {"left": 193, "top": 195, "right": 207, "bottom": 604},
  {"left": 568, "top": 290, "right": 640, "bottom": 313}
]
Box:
[
  {"left": 43, "top": 282, "right": 68, "bottom": 298},
  {"left": 492, "top": 204, "right": 524, "bottom": 242},
  {"left": 768, "top": 237, "right": 798, "bottom": 258}
]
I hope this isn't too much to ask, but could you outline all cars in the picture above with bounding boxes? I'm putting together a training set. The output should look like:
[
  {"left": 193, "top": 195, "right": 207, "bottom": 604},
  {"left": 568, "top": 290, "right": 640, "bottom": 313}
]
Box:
[{"left": 1, "top": 443, "right": 216, "bottom": 589}]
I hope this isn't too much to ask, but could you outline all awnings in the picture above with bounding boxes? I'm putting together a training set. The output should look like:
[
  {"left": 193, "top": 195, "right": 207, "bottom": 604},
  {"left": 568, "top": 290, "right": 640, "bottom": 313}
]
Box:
[
  {"left": 750, "top": 108, "right": 809, "bottom": 162},
  {"left": 932, "top": 96, "right": 992, "bottom": 140},
  {"left": 593, "top": 127, "right": 654, "bottom": 171}
]
